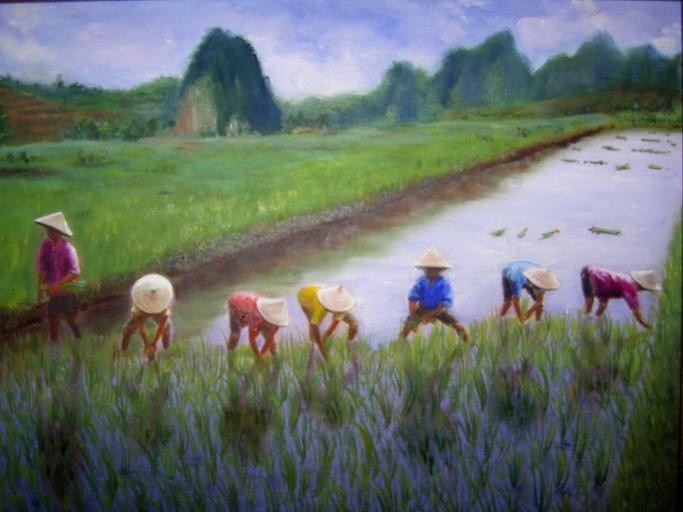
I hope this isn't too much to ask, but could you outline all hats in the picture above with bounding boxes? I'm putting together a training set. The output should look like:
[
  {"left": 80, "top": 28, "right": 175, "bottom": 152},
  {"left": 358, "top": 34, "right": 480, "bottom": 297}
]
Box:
[
  {"left": 414, "top": 248, "right": 451, "bottom": 269},
  {"left": 317, "top": 285, "right": 355, "bottom": 313},
  {"left": 523, "top": 268, "right": 560, "bottom": 290},
  {"left": 131, "top": 274, "right": 174, "bottom": 314},
  {"left": 256, "top": 297, "right": 289, "bottom": 326},
  {"left": 34, "top": 211, "right": 73, "bottom": 237},
  {"left": 631, "top": 270, "right": 661, "bottom": 291}
]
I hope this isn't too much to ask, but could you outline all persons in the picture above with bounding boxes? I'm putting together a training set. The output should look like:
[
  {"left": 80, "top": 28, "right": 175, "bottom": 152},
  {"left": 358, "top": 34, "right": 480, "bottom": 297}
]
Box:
[
  {"left": 225, "top": 291, "right": 288, "bottom": 357},
  {"left": 30, "top": 212, "right": 84, "bottom": 341},
  {"left": 296, "top": 283, "right": 360, "bottom": 359},
  {"left": 120, "top": 272, "right": 175, "bottom": 356},
  {"left": 399, "top": 247, "right": 468, "bottom": 344},
  {"left": 498, "top": 260, "right": 558, "bottom": 324},
  {"left": 580, "top": 265, "right": 660, "bottom": 331}
]
